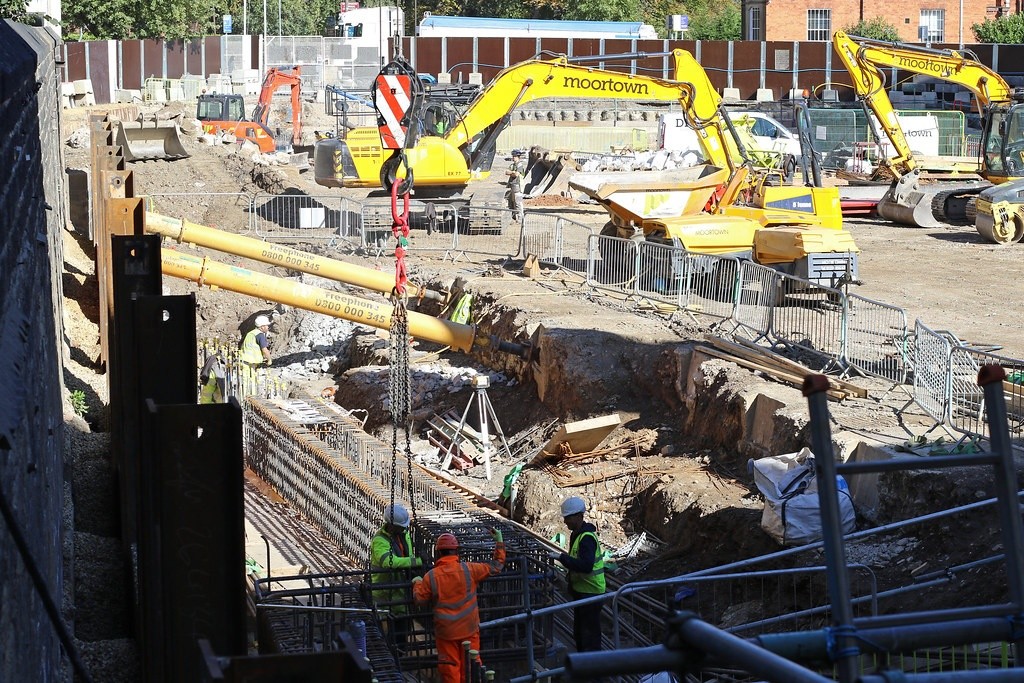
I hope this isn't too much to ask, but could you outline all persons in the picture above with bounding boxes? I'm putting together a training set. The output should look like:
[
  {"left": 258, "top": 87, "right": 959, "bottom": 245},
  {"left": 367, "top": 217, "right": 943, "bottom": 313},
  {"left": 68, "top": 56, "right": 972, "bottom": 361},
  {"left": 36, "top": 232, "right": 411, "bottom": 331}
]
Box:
[
  {"left": 548, "top": 497, "right": 608, "bottom": 656},
  {"left": 369, "top": 502, "right": 427, "bottom": 660},
  {"left": 504, "top": 147, "right": 524, "bottom": 227},
  {"left": 436, "top": 113, "right": 446, "bottom": 135},
  {"left": 411, "top": 526, "right": 507, "bottom": 683},
  {"left": 240, "top": 315, "right": 272, "bottom": 378}
]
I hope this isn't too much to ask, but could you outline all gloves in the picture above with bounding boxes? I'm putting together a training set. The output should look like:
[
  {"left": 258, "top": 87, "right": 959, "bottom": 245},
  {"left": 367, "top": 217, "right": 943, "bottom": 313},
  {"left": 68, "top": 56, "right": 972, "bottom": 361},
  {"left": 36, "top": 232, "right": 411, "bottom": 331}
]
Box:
[
  {"left": 411, "top": 576, "right": 422, "bottom": 582},
  {"left": 266, "top": 359, "right": 272, "bottom": 366},
  {"left": 490, "top": 527, "right": 503, "bottom": 542},
  {"left": 549, "top": 551, "right": 559, "bottom": 559}
]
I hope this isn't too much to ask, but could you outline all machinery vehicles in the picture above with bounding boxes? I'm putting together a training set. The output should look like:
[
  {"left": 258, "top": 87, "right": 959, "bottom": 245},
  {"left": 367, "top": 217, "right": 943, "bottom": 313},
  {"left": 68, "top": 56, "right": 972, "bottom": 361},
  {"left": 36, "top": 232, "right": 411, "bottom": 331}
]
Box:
[
  {"left": 195, "top": 63, "right": 303, "bottom": 155},
  {"left": 834, "top": 30, "right": 1024, "bottom": 245},
  {"left": 308, "top": 48, "right": 860, "bottom": 306}
]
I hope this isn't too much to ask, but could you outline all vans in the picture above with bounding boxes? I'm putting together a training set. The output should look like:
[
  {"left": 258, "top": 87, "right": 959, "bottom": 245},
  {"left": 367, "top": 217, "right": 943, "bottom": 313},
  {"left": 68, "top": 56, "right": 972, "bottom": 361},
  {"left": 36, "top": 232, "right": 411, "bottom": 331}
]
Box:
[{"left": 663, "top": 108, "right": 801, "bottom": 178}]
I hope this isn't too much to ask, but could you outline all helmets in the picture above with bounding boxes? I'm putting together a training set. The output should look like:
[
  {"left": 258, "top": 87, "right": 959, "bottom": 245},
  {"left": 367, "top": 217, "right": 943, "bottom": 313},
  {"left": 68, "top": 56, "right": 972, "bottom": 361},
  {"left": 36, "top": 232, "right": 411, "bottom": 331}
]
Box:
[
  {"left": 382, "top": 505, "right": 410, "bottom": 527},
  {"left": 435, "top": 533, "right": 458, "bottom": 550},
  {"left": 255, "top": 315, "right": 271, "bottom": 327},
  {"left": 561, "top": 497, "right": 585, "bottom": 517}
]
